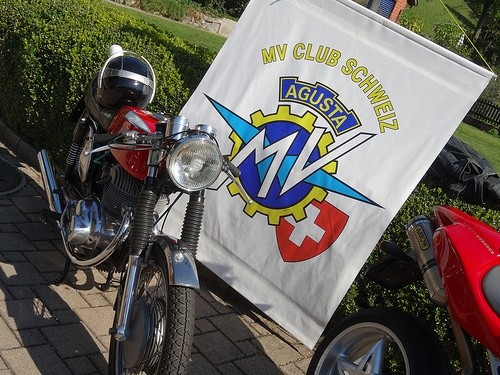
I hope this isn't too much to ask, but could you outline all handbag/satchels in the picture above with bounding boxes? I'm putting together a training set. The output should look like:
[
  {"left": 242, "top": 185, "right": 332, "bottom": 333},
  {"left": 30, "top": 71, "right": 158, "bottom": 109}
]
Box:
[{"left": 427, "top": 134, "right": 499, "bottom": 212}]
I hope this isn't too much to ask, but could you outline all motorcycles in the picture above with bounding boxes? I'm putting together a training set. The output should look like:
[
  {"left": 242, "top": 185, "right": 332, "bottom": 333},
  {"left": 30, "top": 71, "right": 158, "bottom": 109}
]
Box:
[
  {"left": 303, "top": 205, "right": 500, "bottom": 374},
  {"left": 37, "top": 44, "right": 253, "bottom": 375}
]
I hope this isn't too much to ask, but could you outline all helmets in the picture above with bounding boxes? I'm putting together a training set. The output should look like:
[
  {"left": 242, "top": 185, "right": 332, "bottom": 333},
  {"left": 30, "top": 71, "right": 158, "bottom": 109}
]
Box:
[{"left": 96, "top": 51, "right": 156, "bottom": 111}]
